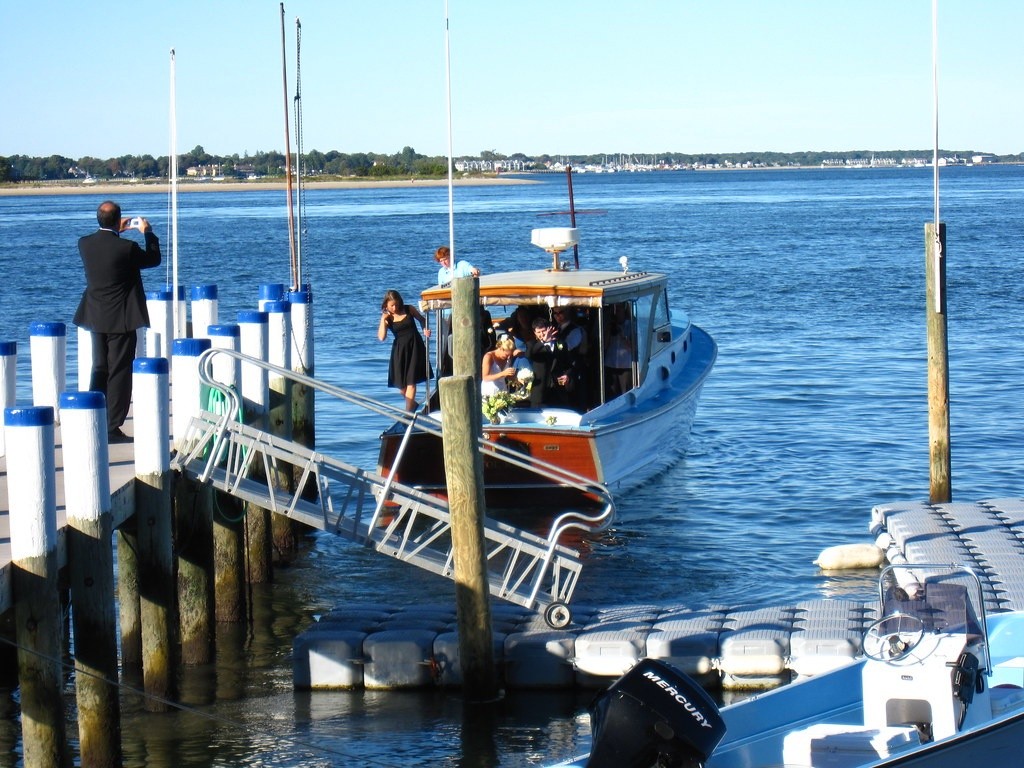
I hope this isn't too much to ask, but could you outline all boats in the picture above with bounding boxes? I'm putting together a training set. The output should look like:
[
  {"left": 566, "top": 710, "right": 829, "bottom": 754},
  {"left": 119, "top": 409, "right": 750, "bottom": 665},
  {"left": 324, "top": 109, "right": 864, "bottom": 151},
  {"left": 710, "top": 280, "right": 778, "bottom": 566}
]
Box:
[
  {"left": 550, "top": 563, "right": 1024, "bottom": 768},
  {"left": 376, "top": 0, "right": 718, "bottom": 526}
]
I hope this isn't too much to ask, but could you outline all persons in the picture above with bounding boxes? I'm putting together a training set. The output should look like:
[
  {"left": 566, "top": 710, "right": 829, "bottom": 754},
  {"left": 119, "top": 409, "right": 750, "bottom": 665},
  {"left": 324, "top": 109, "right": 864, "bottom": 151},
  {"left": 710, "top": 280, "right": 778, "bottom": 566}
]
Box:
[
  {"left": 378, "top": 289, "right": 433, "bottom": 427},
  {"left": 72, "top": 200, "right": 162, "bottom": 444},
  {"left": 434, "top": 247, "right": 479, "bottom": 335},
  {"left": 479, "top": 304, "right": 635, "bottom": 416}
]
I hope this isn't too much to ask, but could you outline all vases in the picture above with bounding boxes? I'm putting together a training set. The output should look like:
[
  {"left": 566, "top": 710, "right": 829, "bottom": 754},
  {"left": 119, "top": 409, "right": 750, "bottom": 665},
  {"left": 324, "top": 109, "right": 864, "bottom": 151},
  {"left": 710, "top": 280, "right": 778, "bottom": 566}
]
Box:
[{"left": 491, "top": 414, "right": 500, "bottom": 425}]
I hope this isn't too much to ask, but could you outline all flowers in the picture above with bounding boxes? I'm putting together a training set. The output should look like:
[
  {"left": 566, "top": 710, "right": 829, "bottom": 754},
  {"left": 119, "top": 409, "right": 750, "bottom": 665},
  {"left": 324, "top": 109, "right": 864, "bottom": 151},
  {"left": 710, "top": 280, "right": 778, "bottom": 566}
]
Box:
[
  {"left": 546, "top": 416, "right": 557, "bottom": 425},
  {"left": 558, "top": 344, "right": 563, "bottom": 350},
  {"left": 482, "top": 394, "right": 515, "bottom": 419}
]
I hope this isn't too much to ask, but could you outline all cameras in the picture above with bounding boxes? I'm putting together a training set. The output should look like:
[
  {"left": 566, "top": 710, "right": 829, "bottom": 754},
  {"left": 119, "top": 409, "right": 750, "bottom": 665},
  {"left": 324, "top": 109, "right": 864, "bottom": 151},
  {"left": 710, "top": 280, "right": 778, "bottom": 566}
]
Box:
[{"left": 131, "top": 220, "right": 140, "bottom": 227}]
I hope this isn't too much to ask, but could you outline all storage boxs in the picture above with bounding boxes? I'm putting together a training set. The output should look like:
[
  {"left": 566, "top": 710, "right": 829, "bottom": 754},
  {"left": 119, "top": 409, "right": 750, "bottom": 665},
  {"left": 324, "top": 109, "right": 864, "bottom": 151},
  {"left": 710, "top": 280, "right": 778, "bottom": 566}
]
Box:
[{"left": 783, "top": 725, "right": 919, "bottom": 768}]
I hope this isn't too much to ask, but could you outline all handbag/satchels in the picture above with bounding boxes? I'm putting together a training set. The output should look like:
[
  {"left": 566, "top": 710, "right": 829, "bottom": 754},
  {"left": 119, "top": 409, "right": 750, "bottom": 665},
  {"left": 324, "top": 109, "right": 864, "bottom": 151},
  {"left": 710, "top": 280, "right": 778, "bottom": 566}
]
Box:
[{"left": 572, "top": 379, "right": 593, "bottom": 411}]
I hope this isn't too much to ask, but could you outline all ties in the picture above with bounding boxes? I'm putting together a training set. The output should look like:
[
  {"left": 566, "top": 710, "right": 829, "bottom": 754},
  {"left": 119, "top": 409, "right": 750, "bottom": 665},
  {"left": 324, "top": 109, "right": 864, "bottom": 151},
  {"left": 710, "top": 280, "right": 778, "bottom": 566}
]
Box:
[{"left": 558, "top": 325, "right": 561, "bottom": 334}]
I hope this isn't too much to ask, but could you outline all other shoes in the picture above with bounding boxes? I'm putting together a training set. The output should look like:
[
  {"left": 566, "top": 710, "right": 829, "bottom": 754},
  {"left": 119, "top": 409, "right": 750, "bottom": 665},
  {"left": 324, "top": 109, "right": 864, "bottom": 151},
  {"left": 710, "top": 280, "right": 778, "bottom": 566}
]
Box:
[
  {"left": 108, "top": 432, "right": 134, "bottom": 444},
  {"left": 402, "top": 416, "right": 410, "bottom": 428}
]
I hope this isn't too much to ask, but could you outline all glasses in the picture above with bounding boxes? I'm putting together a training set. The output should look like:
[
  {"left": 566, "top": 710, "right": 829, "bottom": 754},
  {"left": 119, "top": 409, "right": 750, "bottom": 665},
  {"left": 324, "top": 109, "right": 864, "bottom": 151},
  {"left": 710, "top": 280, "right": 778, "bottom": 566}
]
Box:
[{"left": 552, "top": 310, "right": 564, "bottom": 315}]
